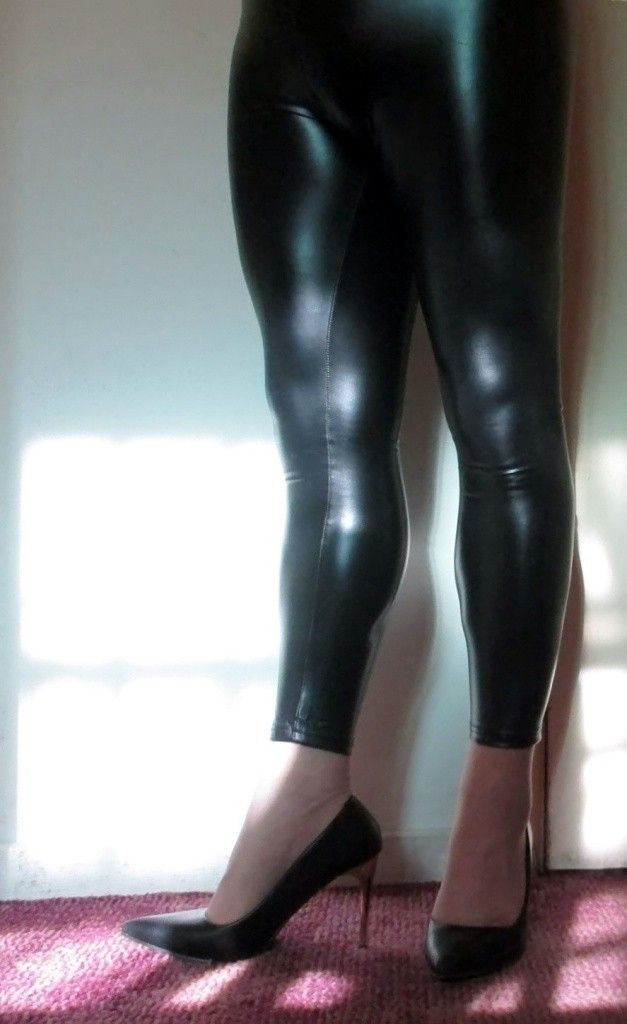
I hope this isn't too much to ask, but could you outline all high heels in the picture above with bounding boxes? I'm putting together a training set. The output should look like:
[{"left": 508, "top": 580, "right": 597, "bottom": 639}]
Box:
[
  {"left": 119, "top": 792, "right": 383, "bottom": 963},
  {"left": 424, "top": 817, "right": 534, "bottom": 980}
]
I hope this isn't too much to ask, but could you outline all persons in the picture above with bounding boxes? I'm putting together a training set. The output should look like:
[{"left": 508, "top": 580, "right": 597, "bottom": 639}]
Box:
[{"left": 123, "top": 0, "right": 579, "bottom": 989}]
